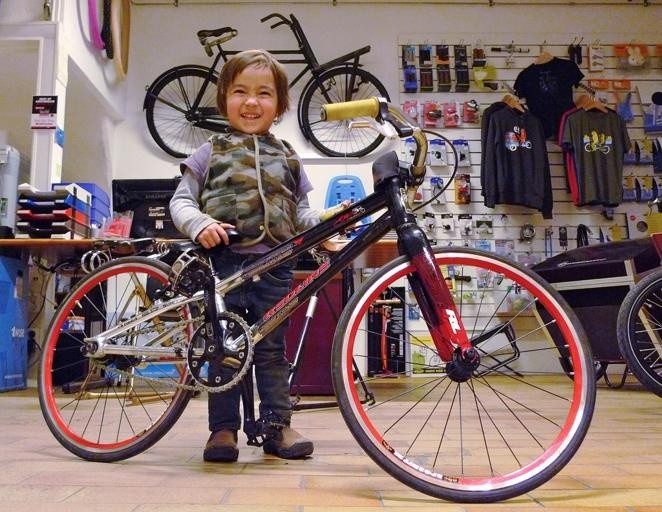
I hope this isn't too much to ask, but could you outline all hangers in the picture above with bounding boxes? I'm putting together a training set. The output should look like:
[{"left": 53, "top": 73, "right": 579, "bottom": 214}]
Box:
[{"left": 503, "top": 42, "right": 607, "bottom": 112}]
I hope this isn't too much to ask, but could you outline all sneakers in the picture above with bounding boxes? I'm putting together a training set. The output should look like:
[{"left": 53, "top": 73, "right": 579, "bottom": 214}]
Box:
[
  {"left": 263, "top": 427, "right": 314, "bottom": 458},
  {"left": 203, "top": 430, "right": 239, "bottom": 463}
]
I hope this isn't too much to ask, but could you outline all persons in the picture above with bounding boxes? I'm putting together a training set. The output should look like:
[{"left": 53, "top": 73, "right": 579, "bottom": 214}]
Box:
[{"left": 169, "top": 49, "right": 357, "bottom": 463}]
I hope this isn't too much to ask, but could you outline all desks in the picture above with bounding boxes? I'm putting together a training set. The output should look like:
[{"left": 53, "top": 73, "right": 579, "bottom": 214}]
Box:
[{"left": 1, "top": 236, "right": 342, "bottom": 404}]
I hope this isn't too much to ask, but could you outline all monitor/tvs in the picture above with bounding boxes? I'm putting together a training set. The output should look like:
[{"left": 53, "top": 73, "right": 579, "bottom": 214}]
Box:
[{"left": 112, "top": 179, "right": 190, "bottom": 239}]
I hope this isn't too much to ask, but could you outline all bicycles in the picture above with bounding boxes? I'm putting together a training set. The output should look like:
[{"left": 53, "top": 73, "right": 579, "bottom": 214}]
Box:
[
  {"left": 616, "top": 268, "right": 662, "bottom": 399},
  {"left": 142, "top": 13, "right": 391, "bottom": 158},
  {"left": 37, "top": 98, "right": 597, "bottom": 504}
]
